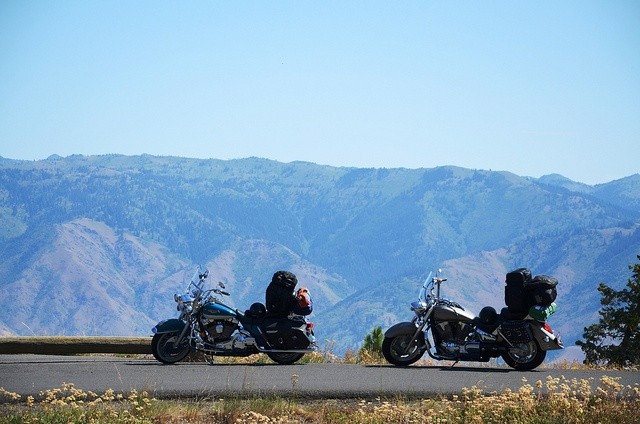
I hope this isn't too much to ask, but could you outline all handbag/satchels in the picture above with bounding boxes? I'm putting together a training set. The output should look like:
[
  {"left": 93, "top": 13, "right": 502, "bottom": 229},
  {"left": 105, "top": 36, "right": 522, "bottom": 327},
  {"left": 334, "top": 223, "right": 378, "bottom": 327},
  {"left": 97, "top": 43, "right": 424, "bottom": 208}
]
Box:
[
  {"left": 528, "top": 302, "right": 556, "bottom": 321},
  {"left": 504, "top": 268, "right": 532, "bottom": 308},
  {"left": 265, "top": 271, "right": 298, "bottom": 313},
  {"left": 297, "top": 288, "right": 310, "bottom": 307},
  {"left": 531, "top": 275, "right": 558, "bottom": 306}
]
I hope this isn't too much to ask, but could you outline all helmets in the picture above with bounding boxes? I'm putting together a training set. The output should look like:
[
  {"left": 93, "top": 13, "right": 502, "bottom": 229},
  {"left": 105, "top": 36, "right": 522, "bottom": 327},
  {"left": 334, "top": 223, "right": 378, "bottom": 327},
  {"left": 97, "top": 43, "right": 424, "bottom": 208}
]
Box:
[
  {"left": 479, "top": 306, "right": 498, "bottom": 325},
  {"left": 249, "top": 303, "right": 266, "bottom": 319}
]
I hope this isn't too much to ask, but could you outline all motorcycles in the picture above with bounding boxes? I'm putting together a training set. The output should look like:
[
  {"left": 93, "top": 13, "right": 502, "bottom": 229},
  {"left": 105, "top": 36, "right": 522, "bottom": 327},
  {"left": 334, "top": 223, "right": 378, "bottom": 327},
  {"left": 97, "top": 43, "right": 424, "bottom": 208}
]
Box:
[
  {"left": 382, "top": 267, "right": 565, "bottom": 371},
  {"left": 151, "top": 265, "right": 316, "bottom": 364}
]
